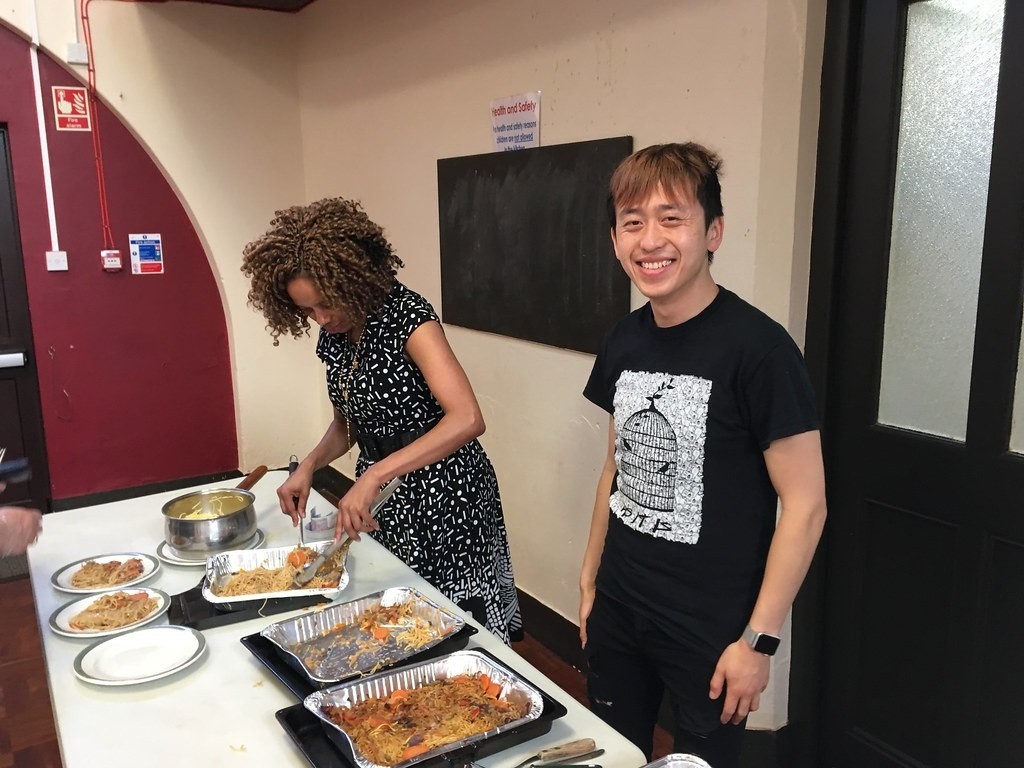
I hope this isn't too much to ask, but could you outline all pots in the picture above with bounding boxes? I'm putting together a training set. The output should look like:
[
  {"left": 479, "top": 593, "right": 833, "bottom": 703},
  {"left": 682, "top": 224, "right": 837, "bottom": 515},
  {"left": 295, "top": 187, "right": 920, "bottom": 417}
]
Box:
[{"left": 161, "top": 466, "right": 268, "bottom": 560}]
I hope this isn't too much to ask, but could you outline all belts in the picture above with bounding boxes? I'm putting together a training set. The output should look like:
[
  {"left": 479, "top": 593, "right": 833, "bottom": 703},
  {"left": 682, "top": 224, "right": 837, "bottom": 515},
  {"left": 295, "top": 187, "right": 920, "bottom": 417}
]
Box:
[{"left": 356, "top": 419, "right": 440, "bottom": 462}]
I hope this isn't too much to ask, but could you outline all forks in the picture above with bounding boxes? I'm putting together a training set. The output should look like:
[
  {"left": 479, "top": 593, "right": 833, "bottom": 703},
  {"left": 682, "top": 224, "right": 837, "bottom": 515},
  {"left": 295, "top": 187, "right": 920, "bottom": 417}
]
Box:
[{"left": 469, "top": 761, "right": 604, "bottom": 768}]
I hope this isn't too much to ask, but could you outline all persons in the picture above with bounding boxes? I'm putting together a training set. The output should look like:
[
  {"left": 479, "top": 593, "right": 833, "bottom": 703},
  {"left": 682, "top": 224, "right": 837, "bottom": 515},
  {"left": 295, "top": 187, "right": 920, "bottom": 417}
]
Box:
[
  {"left": 0, "top": 506, "right": 43, "bottom": 560},
  {"left": 577, "top": 142, "right": 831, "bottom": 768},
  {"left": 241, "top": 196, "right": 524, "bottom": 648}
]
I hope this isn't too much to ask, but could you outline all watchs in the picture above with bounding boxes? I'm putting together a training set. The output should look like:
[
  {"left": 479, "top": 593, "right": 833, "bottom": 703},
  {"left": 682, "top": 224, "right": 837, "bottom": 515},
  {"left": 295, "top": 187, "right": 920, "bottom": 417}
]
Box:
[{"left": 742, "top": 624, "right": 783, "bottom": 657}]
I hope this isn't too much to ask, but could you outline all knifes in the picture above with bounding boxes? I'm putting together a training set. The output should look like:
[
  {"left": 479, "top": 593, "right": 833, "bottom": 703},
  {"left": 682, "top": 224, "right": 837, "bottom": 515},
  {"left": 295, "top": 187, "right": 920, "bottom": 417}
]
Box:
[{"left": 288, "top": 454, "right": 304, "bottom": 543}]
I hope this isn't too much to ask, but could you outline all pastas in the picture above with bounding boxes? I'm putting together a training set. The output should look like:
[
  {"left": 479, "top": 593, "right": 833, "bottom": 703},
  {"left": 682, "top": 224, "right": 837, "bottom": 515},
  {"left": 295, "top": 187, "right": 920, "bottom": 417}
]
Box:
[
  {"left": 70, "top": 558, "right": 141, "bottom": 586},
  {"left": 178, "top": 495, "right": 242, "bottom": 520},
  {"left": 393, "top": 603, "right": 442, "bottom": 651},
  {"left": 219, "top": 563, "right": 326, "bottom": 597},
  {"left": 356, "top": 683, "right": 518, "bottom": 767},
  {"left": 70, "top": 593, "right": 156, "bottom": 629}
]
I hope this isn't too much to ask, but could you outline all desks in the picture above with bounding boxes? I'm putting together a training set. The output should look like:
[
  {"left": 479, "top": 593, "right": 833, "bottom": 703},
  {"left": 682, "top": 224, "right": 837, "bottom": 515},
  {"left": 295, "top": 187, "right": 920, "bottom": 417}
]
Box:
[{"left": 27, "top": 473, "right": 647, "bottom": 768}]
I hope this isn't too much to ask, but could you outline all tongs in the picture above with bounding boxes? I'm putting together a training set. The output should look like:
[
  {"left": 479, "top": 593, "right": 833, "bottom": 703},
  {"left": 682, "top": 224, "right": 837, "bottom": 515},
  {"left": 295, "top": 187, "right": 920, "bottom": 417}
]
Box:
[{"left": 294, "top": 476, "right": 402, "bottom": 587}]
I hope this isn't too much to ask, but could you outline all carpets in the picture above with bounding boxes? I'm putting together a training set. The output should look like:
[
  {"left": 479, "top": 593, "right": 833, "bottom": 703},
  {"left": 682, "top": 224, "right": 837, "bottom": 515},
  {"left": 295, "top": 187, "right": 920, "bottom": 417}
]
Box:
[{"left": 0, "top": 553, "right": 29, "bottom": 583}]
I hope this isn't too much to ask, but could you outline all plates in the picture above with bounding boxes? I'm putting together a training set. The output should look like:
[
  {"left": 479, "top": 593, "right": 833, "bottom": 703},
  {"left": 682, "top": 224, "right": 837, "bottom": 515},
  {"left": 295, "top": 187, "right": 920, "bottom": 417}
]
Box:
[
  {"left": 72, "top": 625, "right": 207, "bottom": 686},
  {"left": 275, "top": 648, "right": 566, "bottom": 768},
  {"left": 167, "top": 585, "right": 332, "bottom": 629},
  {"left": 50, "top": 552, "right": 160, "bottom": 594},
  {"left": 241, "top": 592, "right": 478, "bottom": 698},
  {"left": 50, "top": 588, "right": 172, "bottom": 638},
  {"left": 155, "top": 527, "right": 265, "bottom": 566}
]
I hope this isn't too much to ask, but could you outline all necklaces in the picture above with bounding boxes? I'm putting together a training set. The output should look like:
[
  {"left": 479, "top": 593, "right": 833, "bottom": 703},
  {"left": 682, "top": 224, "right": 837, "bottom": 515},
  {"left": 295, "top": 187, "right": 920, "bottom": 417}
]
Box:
[{"left": 338, "top": 318, "right": 368, "bottom": 460}]
